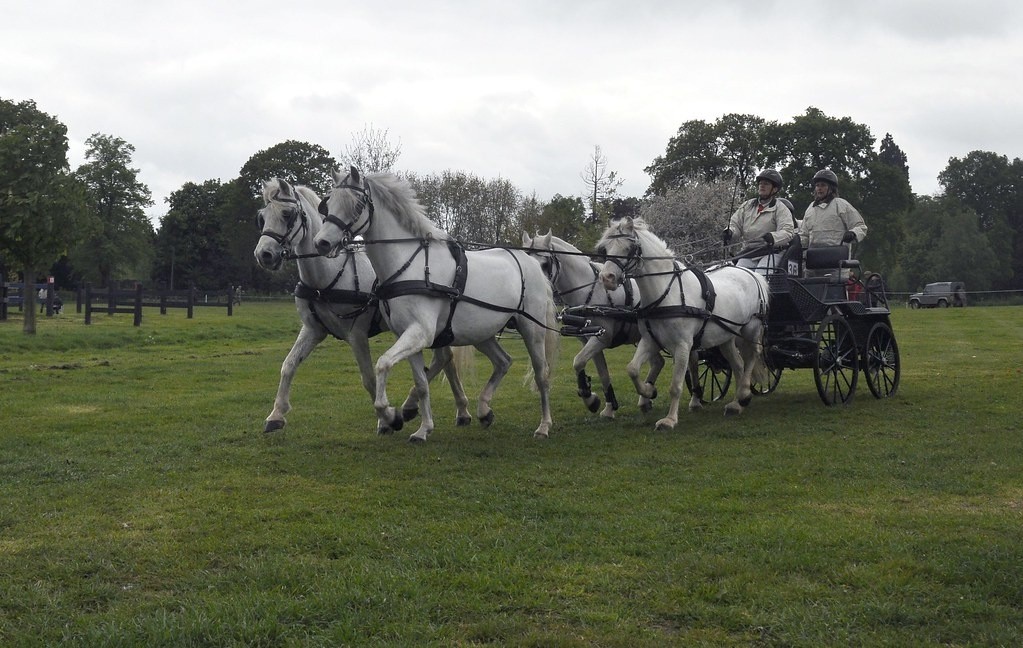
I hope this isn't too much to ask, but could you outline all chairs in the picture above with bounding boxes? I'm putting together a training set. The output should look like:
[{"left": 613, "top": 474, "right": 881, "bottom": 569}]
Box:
[
  {"left": 800, "top": 243, "right": 853, "bottom": 285},
  {"left": 764, "top": 197, "right": 802, "bottom": 276}
]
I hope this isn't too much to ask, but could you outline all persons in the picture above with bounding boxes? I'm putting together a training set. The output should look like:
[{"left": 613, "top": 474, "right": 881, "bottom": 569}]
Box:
[
  {"left": 38, "top": 288, "right": 49, "bottom": 313},
  {"left": 228, "top": 285, "right": 236, "bottom": 304},
  {"left": 849, "top": 269, "right": 861, "bottom": 300},
  {"left": 53, "top": 293, "right": 63, "bottom": 314},
  {"left": 864, "top": 270, "right": 883, "bottom": 307},
  {"left": 233, "top": 285, "right": 245, "bottom": 306},
  {"left": 958, "top": 286, "right": 968, "bottom": 307},
  {"left": 722, "top": 170, "right": 793, "bottom": 286},
  {"left": 793, "top": 169, "right": 867, "bottom": 345}
]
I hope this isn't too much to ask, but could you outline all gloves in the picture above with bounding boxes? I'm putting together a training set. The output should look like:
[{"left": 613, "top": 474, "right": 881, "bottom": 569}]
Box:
[
  {"left": 842, "top": 230, "right": 856, "bottom": 243},
  {"left": 762, "top": 232, "right": 775, "bottom": 245},
  {"left": 721, "top": 229, "right": 733, "bottom": 241}
]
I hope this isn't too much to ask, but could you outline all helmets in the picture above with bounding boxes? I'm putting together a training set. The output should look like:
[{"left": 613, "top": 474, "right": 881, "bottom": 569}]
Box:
[
  {"left": 811, "top": 169, "right": 840, "bottom": 186},
  {"left": 755, "top": 169, "right": 785, "bottom": 188}
]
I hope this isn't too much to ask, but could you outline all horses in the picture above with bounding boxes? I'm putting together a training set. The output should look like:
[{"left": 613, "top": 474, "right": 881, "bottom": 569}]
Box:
[
  {"left": 251, "top": 175, "right": 473, "bottom": 436},
  {"left": 519, "top": 229, "right": 704, "bottom": 416},
  {"left": 598, "top": 216, "right": 768, "bottom": 430},
  {"left": 313, "top": 165, "right": 559, "bottom": 445}
]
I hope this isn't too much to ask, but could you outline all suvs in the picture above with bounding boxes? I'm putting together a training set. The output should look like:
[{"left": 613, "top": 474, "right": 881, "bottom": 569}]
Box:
[{"left": 908, "top": 281, "right": 967, "bottom": 308}]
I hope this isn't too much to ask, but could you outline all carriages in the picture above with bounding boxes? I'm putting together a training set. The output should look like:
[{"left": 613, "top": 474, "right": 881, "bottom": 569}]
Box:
[{"left": 254, "top": 165, "right": 901, "bottom": 450}]
